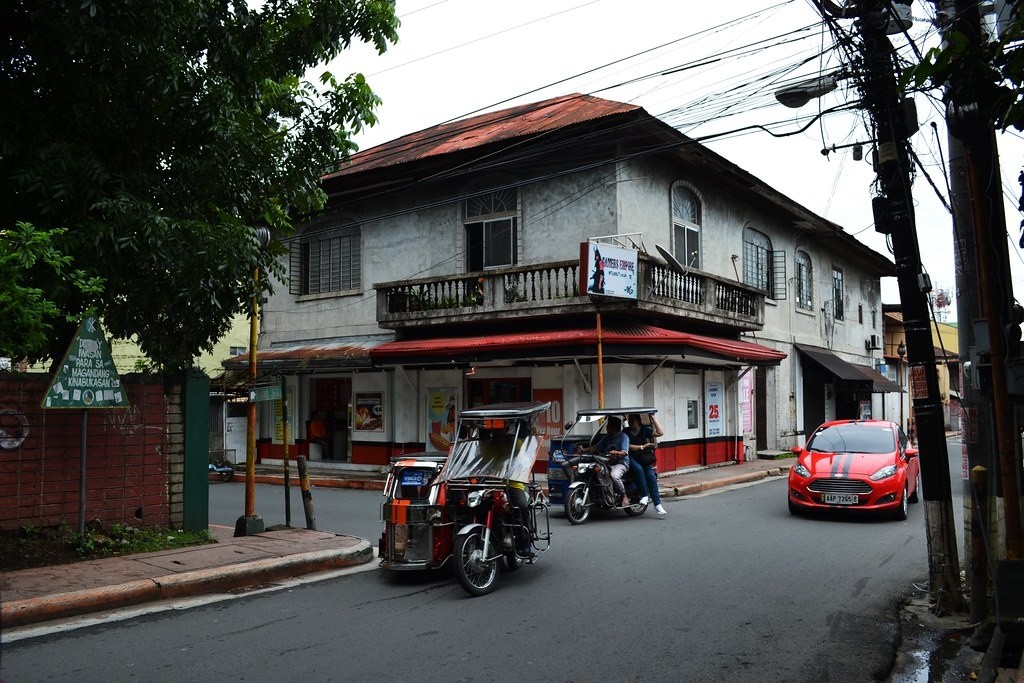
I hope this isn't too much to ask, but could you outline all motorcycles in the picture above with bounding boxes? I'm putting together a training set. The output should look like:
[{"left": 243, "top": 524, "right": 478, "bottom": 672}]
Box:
[
  {"left": 547, "top": 407, "right": 658, "bottom": 525},
  {"left": 378, "top": 401, "right": 554, "bottom": 595}
]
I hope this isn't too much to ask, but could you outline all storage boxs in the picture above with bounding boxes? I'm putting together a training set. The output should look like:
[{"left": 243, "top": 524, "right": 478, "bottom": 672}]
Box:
[{"left": 388, "top": 293, "right": 408, "bottom": 313}]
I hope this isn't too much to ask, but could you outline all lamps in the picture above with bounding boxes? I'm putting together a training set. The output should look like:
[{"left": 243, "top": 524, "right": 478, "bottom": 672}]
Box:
[
  {"left": 867, "top": 334, "right": 882, "bottom": 350},
  {"left": 684, "top": 250, "right": 699, "bottom": 276},
  {"left": 731, "top": 254, "right": 740, "bottom": 282}
]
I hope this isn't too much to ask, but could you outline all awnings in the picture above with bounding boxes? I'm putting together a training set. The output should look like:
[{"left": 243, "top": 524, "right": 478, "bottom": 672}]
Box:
[{"left": 793, "top": 342, "right": 908, "bottom": 393}]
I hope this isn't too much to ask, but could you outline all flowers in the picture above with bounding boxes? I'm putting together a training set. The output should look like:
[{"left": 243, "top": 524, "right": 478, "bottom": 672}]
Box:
[{"left": 466, "top": 278, "right": 484, "bottom": 305}]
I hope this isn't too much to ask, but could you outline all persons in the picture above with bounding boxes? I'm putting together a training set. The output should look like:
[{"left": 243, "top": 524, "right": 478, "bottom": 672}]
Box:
[
  {"left": 310, "top": 411, "right": 338, "bottom": 460},
  {"left": 465, "top": 419, "right": 534, "bottom": 558},
  {"left": 622, "top": 413, "right": 667, "bottom": 515},
  {"left": 474, "top": 277, "right": 485, "bottom": 306},
  {"left": 576, "top": 416, "right": 630, "bottom": 507}
]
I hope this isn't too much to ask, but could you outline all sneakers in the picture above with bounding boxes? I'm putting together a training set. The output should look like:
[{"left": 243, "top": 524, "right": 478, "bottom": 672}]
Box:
[
  {"left": 654, "top": 504, "right": 666, "bottom": 515},
  {"left": 639, "top": 496, "right": 648, "bottom": 505}
]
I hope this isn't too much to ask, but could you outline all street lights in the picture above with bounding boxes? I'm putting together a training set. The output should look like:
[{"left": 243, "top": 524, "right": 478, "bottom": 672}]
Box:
[
  {"left": 897, "top": 340, "right": 906, "bottom": 429},
  {"left": 775, "top": 72, "right": 964, "bottom": 617}
]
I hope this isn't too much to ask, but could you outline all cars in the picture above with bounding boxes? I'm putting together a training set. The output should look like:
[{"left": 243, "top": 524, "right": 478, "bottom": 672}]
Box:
[{"left": 788, "top": 420, "right": 919, "bottom": 520}]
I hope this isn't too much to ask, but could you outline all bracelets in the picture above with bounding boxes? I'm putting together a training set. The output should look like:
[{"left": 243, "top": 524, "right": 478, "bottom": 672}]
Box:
[{"left": 641, "top": 445, "right": 643, "bottom": 449}]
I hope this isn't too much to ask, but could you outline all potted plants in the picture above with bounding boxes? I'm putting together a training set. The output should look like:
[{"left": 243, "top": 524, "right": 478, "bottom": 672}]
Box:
[{"left": 411, "top": 288, "right": 428, "bottom": 311}]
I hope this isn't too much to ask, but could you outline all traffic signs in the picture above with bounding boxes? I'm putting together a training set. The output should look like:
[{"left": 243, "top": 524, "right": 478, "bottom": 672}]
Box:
[{"left": 249, "top": 386, "right": 282, "bottom": 402}]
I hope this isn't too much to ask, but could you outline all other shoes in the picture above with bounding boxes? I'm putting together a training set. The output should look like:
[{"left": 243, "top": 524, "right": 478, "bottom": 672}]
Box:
[
  {"left": 622, "top": 497, "right": 629, "bottom": 506},
  {"left": 523, "top": 548, "right": 535, "bottom": 556},
  {"left": 322, "top": 456, "right": 333, "bottom": 460}
]
